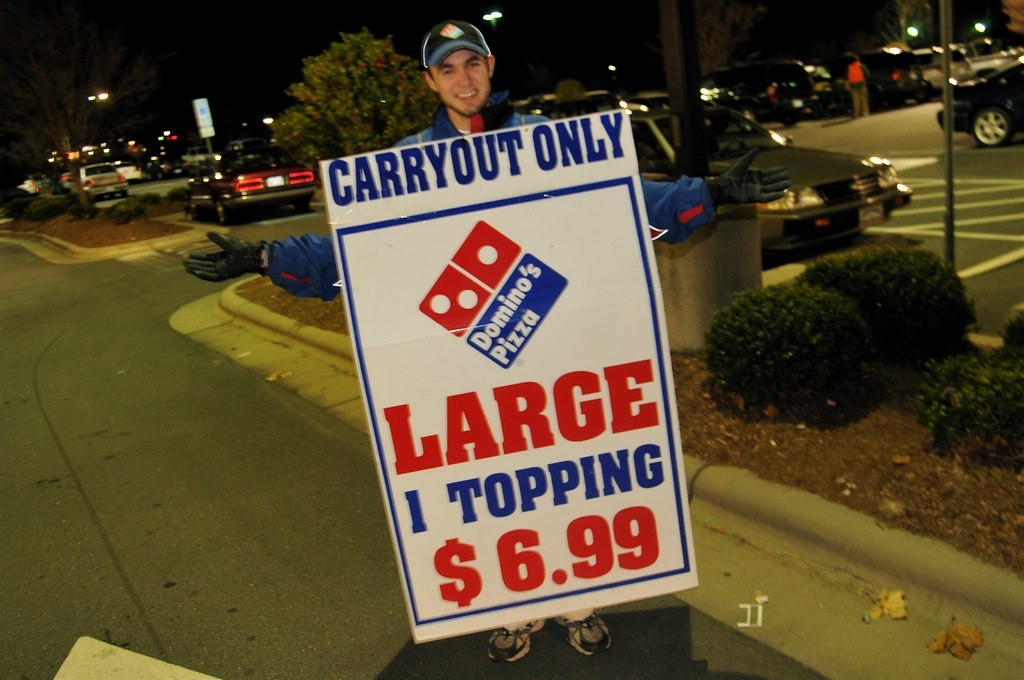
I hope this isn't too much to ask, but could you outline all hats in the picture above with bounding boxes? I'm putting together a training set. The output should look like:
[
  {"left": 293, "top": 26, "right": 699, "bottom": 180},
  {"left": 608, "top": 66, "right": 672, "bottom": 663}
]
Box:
[{"left": 421, "top": 20, "right": 492, "bottom": 69}]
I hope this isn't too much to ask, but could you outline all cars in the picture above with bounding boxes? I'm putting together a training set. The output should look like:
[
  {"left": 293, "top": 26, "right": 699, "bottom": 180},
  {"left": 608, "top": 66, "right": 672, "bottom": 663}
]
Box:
[
  {"left": 17, "top": 137, "right": 268, "bottom": 205},
  {"left": 936, "top": 56, "right": 1024, "bottom": 150},
  {"left": 515, "top": 37, "right": 1023, "bottom": 127},
  {"left": 628, "top": 104, "right": 915, "bottom": 252},
  {"left": 187, "top": 147, "right": 316, "bottom": 225}
]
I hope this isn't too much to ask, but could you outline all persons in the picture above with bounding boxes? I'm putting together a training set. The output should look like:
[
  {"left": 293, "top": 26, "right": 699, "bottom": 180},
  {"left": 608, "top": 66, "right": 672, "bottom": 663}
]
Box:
[
  {"left": 847, "top": 56, "right": 873, "bottom": 117},
  {"left": 181, "top": 23, "right": 794, "bottom": 663}
]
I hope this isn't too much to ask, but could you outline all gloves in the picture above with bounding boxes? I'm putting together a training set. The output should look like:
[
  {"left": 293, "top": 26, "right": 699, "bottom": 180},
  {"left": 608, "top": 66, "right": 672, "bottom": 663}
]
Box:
[
  {"left": 708, "top": 146, "right": 791, "bottom": 207},
  {"left": 181, "top": 232, "right": 267, "bottom": 282}
]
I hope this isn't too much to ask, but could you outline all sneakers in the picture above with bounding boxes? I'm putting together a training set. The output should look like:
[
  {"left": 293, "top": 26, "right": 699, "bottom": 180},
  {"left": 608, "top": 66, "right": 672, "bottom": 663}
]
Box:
[
  {"left": 564, "top": 614, "right": 612, "bottom": 656},
  {"left": 487, "top": 620, "right": 545, "bottom": 659}
]
